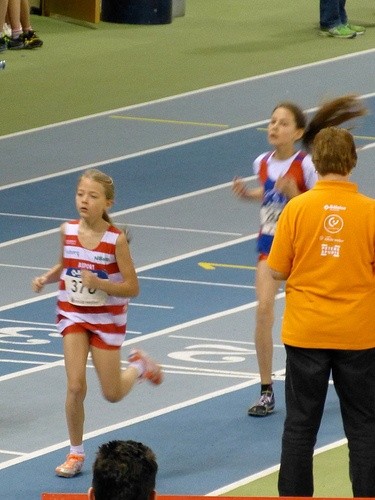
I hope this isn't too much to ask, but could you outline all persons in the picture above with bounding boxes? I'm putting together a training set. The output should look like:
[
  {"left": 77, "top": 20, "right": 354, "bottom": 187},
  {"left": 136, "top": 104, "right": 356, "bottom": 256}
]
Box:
[
  {"left": 87, "top": 439, "right": 158, "bottom": 500},
  {"left": 265, "top": 127, "right": 375, "bottom": 497},
  {"left": 31, "top": 169, "right": 165, "bottom": 478},
  {"left": 231, "top": 93, "right": 369, "bottom": 417},
  {"left": 318, "top": 0, "right": 366, "bottom": 39},
  {"left": 0, "top": 0, "right": 43, "bottom": 69}
]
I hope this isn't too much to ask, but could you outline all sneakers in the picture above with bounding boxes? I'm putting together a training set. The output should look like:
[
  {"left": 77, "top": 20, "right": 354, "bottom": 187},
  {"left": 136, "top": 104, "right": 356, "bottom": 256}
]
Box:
[
  {"left": 127, "top": 350, "right": 163, "bottom": 384},
  {"left": 22, "top": 30, "right": 43, "bottom": 49},
  {"left": 6, "top": 32, "right": 24, "bottom": 50},
  {"left": 55, "top": 454, "right": 85, "bottom": 477},
  {"left": 346, "top": 23, "right": 366, "bottom": 35},
  {"left": 248, "top": 391, "right": 275, "bottom": 415},
  {"left": 0, "top": 37, "right": 7, "bottom": 51},
  {"left": 320, "top": 24, "right": 356, "bottom": 39}
]
